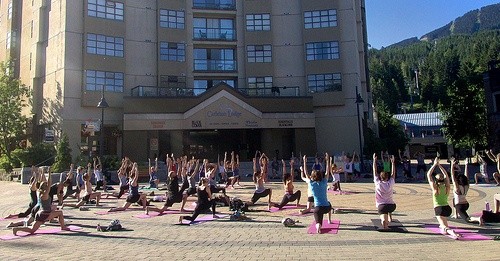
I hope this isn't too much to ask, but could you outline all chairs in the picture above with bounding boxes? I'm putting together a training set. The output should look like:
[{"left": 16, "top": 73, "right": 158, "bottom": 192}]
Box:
[
  {"left": 199, "top": 30, "right": 207, "bottom": 40},
  {"left": 220, "top": 31, "right": 227, "bottom": 40}
]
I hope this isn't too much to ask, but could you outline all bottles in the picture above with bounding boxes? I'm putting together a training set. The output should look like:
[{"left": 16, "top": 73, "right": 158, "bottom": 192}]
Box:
[
  {"left": 24, "top": 217, "right": 27, "bottom": 227},
  {"left": 97, "top": 223, "right": 100, "bottom": 231},
  {"left": 486, "top": 203, "right": 489, "bottom": 210}
]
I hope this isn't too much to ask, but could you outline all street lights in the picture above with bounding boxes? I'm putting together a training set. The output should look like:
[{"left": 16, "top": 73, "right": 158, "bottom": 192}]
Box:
[
  {"left": 355, "top": 85, "right": 365, "bottom": 161},
  {"left": 96, "top": 84, "right": 111, "bottom": 162}
]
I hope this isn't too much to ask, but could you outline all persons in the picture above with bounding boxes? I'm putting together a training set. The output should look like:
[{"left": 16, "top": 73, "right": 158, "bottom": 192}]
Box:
[
  {"left": 451, "top": 158, "right": 483, "bottom": 226},
  {"left": 13, "top": 167, "right": 70, "bottom": 236},
  {"left": 485, "top": 149, "right": 500, "bottom": 186},
  {"left": 427, "top": 157, "right": 462, "bottom": 238},
  {"left": 146, "top": 151, "right": 240, "bottom": 223},
  {"left": 381, "top": 151, "right": 496, "bottom": 184},
  {"left": 493, "top": 194, "right": 500, "bottom": 213},
  {"left": 303, "top": 153, "right": 332, "bottom": 234},
  {"left": 4, "top": 157, "right": 148, "bottom": 228},
  {"left": 373, "top": 153, "right": 397, "bottom": 229},
  {"left": 245, "top": 151, "right": 361, "bottom": 215}
]
